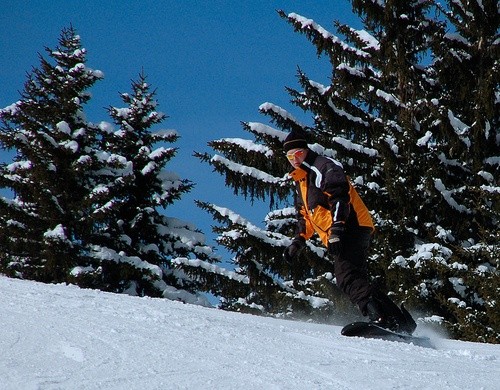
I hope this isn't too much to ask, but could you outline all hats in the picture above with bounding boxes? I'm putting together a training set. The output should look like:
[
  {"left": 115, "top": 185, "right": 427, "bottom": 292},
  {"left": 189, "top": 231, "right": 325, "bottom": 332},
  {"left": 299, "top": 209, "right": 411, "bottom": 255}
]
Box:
[{"left": 283, "top": 131, "right": 306, "bottom": 151}]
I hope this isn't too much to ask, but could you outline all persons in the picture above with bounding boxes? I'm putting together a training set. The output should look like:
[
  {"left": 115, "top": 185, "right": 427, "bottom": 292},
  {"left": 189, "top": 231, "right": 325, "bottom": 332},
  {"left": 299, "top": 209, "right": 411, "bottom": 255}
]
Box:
[{"left": 282, "top": 132, "right": 417, "bottom": 336}]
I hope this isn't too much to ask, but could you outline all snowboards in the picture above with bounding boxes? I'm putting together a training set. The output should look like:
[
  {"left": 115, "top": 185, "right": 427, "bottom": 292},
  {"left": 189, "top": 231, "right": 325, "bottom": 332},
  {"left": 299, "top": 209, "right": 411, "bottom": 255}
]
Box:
[{"left": 341, "top": 322, "right": 430, "bottom": 342}]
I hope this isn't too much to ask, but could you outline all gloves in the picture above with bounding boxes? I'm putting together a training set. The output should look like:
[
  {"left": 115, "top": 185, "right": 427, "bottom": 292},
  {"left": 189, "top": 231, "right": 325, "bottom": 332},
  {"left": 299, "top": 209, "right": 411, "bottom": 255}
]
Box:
[
  {"left": 282, "top": 235, "right": 306, "bottom": 264},
  {"left": 328, "top": 222, "right": 344, "bottom": 254}
]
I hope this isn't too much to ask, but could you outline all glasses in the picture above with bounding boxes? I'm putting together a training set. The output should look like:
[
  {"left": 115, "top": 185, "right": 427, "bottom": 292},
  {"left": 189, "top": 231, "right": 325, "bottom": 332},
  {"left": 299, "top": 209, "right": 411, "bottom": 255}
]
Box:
[{"left": 287, "top": 149, "right": 303, "bottom": 159}]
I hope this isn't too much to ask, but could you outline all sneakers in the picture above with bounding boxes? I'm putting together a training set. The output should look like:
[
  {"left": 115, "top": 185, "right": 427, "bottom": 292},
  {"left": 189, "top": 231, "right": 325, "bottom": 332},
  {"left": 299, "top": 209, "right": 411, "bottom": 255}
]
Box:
[{"left": 367, "top": 316, "right": 413, "bottom": 335}]
